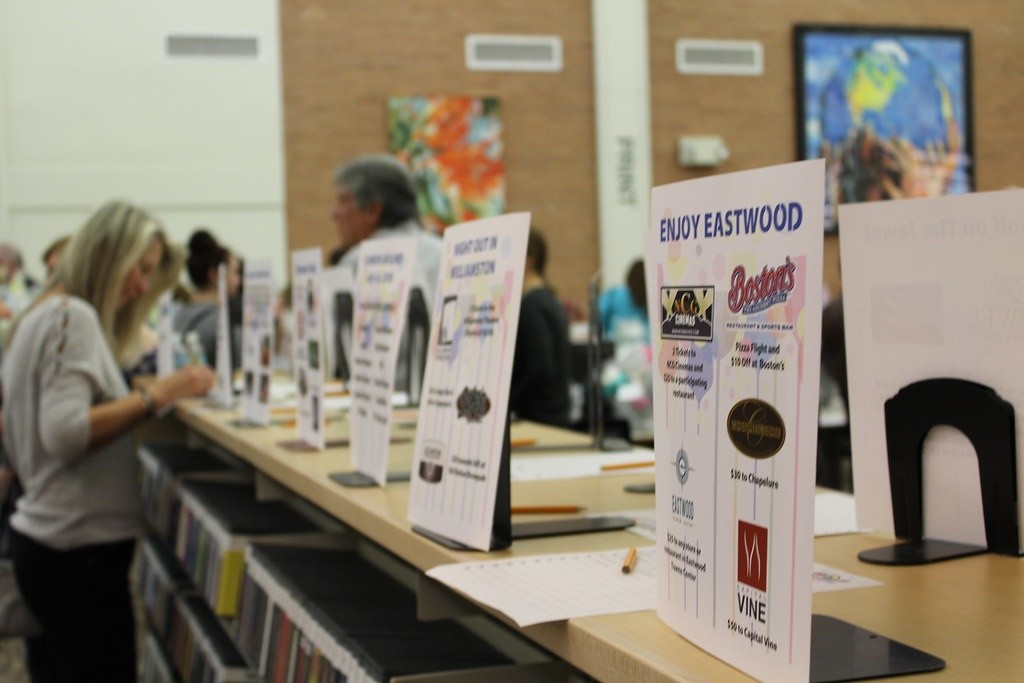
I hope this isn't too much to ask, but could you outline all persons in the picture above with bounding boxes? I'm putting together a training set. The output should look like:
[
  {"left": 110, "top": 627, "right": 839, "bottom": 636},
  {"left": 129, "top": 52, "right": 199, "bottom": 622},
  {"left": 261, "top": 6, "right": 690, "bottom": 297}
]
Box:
[
  {"left": 0, "top": 154, "right": 653, "bottom": 683},
  {"left": 816, "top": 225, "right": 854, "bottom": 493}
]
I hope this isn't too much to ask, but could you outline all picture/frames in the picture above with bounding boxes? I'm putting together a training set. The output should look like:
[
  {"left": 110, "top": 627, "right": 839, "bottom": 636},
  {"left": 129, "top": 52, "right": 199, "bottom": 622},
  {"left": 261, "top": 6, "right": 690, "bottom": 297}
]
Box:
[{"left": 792, "top": 20, "right": 976, "bottom": 235}]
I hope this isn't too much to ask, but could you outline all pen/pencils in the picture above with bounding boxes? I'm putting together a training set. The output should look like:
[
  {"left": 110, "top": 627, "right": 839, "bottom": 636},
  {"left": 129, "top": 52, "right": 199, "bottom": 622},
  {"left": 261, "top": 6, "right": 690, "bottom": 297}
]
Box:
[
  {"left": 509, "top": 438, "right": 536, "bottom": 447},
  {"left": 284, "top": 419, "right": 333, "bottom": 427},
  {"left": 509, "top": 504, "right": 587, "bottom": 515},
  {"left": 183, "top": 341, "right": 199, "bottom": 368},
  {"left": 325, "top": 390, "right": 349, "bottom": 399},
  {"left": 622, "top": 548, "right": 638, "bottom": 573},
  {"left": 601, "top": 461, "right": 657, "bottom": 471}
]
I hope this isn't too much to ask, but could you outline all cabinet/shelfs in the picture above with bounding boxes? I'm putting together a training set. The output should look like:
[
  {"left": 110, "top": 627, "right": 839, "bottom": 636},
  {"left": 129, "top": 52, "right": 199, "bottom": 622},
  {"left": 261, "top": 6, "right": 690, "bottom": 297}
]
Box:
[{"left": 130, "top": 374, "right": 1024, "bottom": 683}]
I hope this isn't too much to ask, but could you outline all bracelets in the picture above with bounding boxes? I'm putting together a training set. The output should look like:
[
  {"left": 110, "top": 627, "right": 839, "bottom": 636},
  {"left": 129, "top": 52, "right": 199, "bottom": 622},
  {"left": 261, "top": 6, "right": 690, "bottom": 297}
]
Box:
[{"left": 138, "top": 387, "right": 156, "bottom": 415}]
